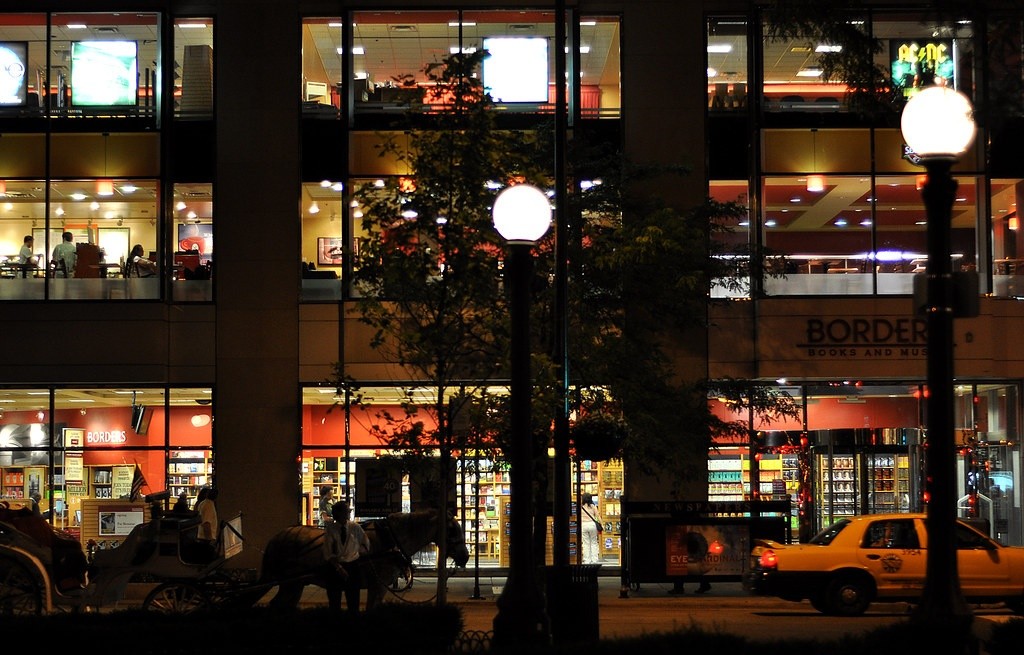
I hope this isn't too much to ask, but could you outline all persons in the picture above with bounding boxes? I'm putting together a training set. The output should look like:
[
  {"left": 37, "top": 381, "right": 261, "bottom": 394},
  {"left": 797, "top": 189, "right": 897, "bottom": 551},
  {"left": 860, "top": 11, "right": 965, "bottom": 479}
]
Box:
[
  {"left": 666, "top": 581, "right": 712, "bottom": 595},
  {"left": 29, "top": 493, "right": 42, "bottom": 513},
  {"left": 322, "top": 501, "right": 370, "bottom": 612},
  {"left": 581, "top": 493, "right": 603, "bottom": 564},
  {"left": 318, "top": 487, "right": 336, "bottom": 530},
  {"left": 174, "top": 488, "right": 219, "bottom": 548},
  {"left": 127, "top": 245, "right": 158, "bottom": 278},
  {"left": 52, "top": 232, "right": 78, "bottom": 278},
  {"left": 17, "top": 235, "right": 39, "bottom": 279}
]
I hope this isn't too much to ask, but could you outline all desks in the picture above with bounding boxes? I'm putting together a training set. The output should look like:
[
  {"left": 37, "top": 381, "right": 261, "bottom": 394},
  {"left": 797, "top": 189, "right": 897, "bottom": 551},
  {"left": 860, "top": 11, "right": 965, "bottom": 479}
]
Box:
[
  {"left": 88, "top": 263, "right": 120, "bottom": 278},
  {"left": 164, "top": 264, "right": 182, "bottom": 279},
  {"left": 994, "top": 258, "right": 1024, "bottom": 275}
]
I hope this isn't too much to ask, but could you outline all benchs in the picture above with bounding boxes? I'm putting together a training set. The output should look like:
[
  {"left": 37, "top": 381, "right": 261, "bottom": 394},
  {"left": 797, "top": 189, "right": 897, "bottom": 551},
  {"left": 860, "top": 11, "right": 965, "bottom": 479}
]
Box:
[{"left": 161, "top": 490, "right": 203, "bottom": 529}]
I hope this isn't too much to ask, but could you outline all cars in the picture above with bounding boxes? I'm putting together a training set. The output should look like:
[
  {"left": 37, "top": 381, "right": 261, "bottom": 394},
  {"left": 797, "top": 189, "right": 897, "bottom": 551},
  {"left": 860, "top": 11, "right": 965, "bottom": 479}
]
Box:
[{"left": 750, "top": 512, "right": 1024, "bottom": 615}]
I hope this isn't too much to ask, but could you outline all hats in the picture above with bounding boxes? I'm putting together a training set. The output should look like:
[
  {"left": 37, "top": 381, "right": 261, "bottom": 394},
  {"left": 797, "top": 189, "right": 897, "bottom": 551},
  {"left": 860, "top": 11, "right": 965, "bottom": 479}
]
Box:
[{"left": 332, "top": 500, "right": 352, "bottom": 515}]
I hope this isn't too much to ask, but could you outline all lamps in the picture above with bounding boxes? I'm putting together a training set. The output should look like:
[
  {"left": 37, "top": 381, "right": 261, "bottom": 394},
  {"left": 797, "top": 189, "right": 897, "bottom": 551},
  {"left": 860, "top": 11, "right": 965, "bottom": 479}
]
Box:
[
  {"left": 32, "top": 219, "right": 38, "bottom": 227},
  {"left": 183, "top": 220, "right": 188, "bottom": 226},
  {"left": 117, "top": 219, "right": 124, "bottom": 226},
  {"left": 958, "top": 388, "right": 963, "bottom": 397},
  {"left": 916, "top": 167, "right": 928, "bottom": 189},
  {"left": 88, "top": 219, "right": 92, "bottom": 226},
  {"left": 61, "top": 219, "right": 66, "bottom": 227},
  {"left": 1009, "top": 218, "right": 1018, "bottom": 231},
  {"left": 805, "top": 129, "right": 824, "bottom": 193},
  {"left": 97, "top": 180, "right": 114, "bottom": 195},
  {"left": 398, "top": 130, "right": 415, "bottom": 195},
  {"left": 149, "top": 219, "right": 155, "bottom": 226},
  {"left": 80, "top": 408, "right": 86, "bottom": 416}
]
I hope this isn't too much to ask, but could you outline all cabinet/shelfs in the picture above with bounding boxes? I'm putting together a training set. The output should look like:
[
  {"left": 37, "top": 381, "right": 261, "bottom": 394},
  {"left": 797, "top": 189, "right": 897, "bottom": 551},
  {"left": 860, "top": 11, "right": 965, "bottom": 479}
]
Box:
[
  {"left": 708, "top": 454, "right": 909, "bottom": 538},
  {"left": 910, "top": 259, "right": 928, "bottom": 273},
  {"left": 302, "top": 456, "right": 624, "bottom": 564},
  {"left": 0, "top": 458, "right": 213, "bottom": 530}
]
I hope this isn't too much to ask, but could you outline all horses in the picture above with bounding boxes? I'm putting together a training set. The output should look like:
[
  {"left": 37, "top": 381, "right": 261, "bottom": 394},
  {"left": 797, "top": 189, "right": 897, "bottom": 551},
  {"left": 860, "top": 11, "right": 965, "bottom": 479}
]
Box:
[{"left": 253, "top": 507, "right": 470, "bottom": 609}]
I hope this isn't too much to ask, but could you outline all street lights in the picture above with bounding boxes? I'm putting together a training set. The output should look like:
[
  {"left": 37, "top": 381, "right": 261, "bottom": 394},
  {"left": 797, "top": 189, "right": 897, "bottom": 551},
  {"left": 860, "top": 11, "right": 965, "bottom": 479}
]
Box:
[
  {"left": 490, "top": 182, "right": 553, "bottom": 647},
  {"left": 900, "top": 85, "right": 977, "bottom": 622}
]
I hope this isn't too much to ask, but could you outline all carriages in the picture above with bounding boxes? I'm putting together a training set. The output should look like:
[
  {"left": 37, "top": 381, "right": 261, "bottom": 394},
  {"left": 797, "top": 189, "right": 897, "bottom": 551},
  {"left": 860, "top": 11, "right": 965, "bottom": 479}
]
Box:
[{"left": 0, "top": 490, "right": 470, "bottom": 617}]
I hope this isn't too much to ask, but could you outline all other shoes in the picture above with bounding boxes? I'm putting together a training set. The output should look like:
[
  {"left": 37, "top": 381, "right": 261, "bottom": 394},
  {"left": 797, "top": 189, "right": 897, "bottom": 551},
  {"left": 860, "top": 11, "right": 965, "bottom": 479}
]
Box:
[
  {"left": 695, "top": 584, "right": 711, "bottom": 593},
  {"left": 69, "top": 588, "right": 86, "bottom": 596},
  {"left": 669, "top": 588, "right": 684, "bottom": 594}
]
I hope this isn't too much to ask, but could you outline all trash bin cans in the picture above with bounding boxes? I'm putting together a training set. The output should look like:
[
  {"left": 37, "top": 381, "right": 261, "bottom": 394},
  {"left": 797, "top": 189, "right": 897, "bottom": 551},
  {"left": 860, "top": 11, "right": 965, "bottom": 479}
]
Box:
[{"left": 542, "top": 563, "right": 601, "bottom": 647}]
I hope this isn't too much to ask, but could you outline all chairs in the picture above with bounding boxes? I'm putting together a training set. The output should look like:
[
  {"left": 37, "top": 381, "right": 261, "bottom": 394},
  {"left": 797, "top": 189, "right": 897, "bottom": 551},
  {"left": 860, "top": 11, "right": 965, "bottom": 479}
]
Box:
[
  {"left": 0, "top": 256, "right": 211, "bottom": 299},
  {"left": 711, "top": 83, "right": 732, "bottom": 107},
  {"left": 730, "top": 83, "right": 746, "bottom": 107}
]
